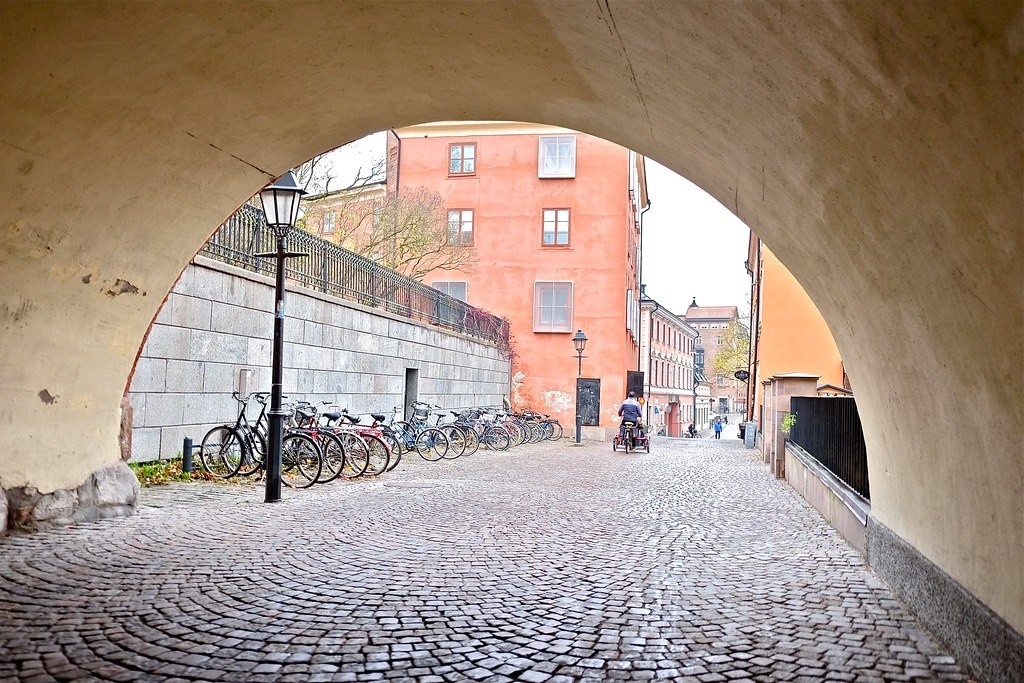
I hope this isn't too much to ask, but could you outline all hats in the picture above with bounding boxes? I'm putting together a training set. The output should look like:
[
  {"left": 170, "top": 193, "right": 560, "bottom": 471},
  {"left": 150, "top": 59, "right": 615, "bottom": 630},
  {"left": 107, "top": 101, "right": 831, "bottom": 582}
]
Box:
[{"left": 629, "top": 391, "right": 635, "bottom": 397}]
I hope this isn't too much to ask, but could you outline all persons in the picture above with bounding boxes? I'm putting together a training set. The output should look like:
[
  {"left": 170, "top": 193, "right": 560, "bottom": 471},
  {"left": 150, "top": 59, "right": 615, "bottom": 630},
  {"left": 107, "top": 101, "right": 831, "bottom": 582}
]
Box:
[
  {"left": 688, "top": 424, "right": 694, "bottom": 438},
  {"left": 724, "top": 416, "right": 728, "bottom": 424},
  {"left": 618, "top": 391, "right": 642, "bottom": 447},
  {"left": 714, "top": 419, "right": 722, "bottom": 439}
]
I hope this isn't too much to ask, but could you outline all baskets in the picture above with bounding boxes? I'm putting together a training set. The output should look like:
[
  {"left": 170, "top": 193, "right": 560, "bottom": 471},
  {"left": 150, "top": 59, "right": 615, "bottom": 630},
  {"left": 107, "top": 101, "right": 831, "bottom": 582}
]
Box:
[
  {"left": 295, "top": 405, "right": 314, "bottom": 425},
  {"left": 694, "top": 429, "right": 698, "bottom": 433},
  {"left": 417, "top": 408, "right": 429, "bottom": 420}
]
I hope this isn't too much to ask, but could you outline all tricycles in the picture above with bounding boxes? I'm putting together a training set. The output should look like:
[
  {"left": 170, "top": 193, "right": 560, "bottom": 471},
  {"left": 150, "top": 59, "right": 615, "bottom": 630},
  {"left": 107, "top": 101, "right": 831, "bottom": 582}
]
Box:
[{"left": 613, "top": 413, "right": 650, "bottom": 454}]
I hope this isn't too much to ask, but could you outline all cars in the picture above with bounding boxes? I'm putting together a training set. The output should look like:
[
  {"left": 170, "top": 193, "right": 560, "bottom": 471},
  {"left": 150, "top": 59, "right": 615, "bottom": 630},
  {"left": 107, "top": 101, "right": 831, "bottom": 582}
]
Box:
[{"left": 710, "top": 411, "right": 719, "bottom": 420}]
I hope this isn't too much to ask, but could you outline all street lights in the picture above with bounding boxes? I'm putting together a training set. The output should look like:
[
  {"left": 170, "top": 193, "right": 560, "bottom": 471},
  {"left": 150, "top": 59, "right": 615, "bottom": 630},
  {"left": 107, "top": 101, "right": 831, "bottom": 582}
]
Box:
[
  {"left": 254, "top": 171, "right": 306, "bottom": 505},
  {"left": 572, "top": 330, "right": 587, "bottom": 442}
]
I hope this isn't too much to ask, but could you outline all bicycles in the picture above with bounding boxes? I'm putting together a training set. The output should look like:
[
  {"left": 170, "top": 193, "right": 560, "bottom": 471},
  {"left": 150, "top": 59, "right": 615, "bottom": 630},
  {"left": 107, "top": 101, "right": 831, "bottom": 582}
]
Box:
[
  {"left": 682, "top": 430, "right": 702, "bottom": 438},
  {"left": 200, "top": 391, "right": 564, "bottom": 490}
]
